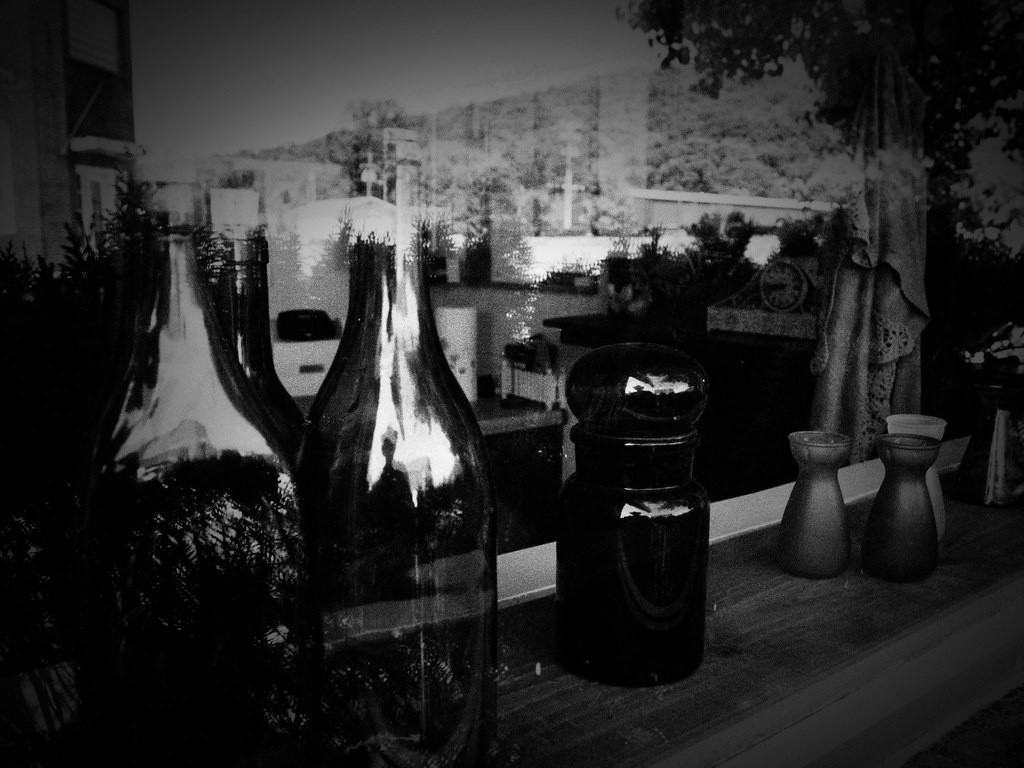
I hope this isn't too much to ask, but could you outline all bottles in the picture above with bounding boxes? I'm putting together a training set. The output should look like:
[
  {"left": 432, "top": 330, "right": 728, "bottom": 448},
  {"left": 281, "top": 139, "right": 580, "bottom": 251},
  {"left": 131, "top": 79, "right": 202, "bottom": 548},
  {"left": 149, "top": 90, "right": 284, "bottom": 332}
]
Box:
[
  {"left": 76, "top": 177, "right": 307, "bottom": 768},
  {"left": 299, "top": 128, "right": 497, "bottom": 768},
  {"left": 202, "top": 236, "right": 307, "bottom": 497},
  {"left": 885, "top": 414, "right": 947, "bottom": 541},
  {"left": 860, "top": 434, "right": 939, "bottom": 582},
  {"left": 552, "top": 340, "right": 710, "bottom": 687},
  {"left": 774, "top": 430, "right": 852, "bottom": 579}
]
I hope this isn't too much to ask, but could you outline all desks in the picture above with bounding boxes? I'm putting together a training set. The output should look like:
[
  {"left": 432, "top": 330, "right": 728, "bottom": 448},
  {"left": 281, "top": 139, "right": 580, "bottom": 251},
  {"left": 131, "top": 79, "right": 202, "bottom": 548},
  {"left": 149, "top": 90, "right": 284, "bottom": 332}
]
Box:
[{"left": 294, "top": 390, "right": 562, "bottom": 494}]
